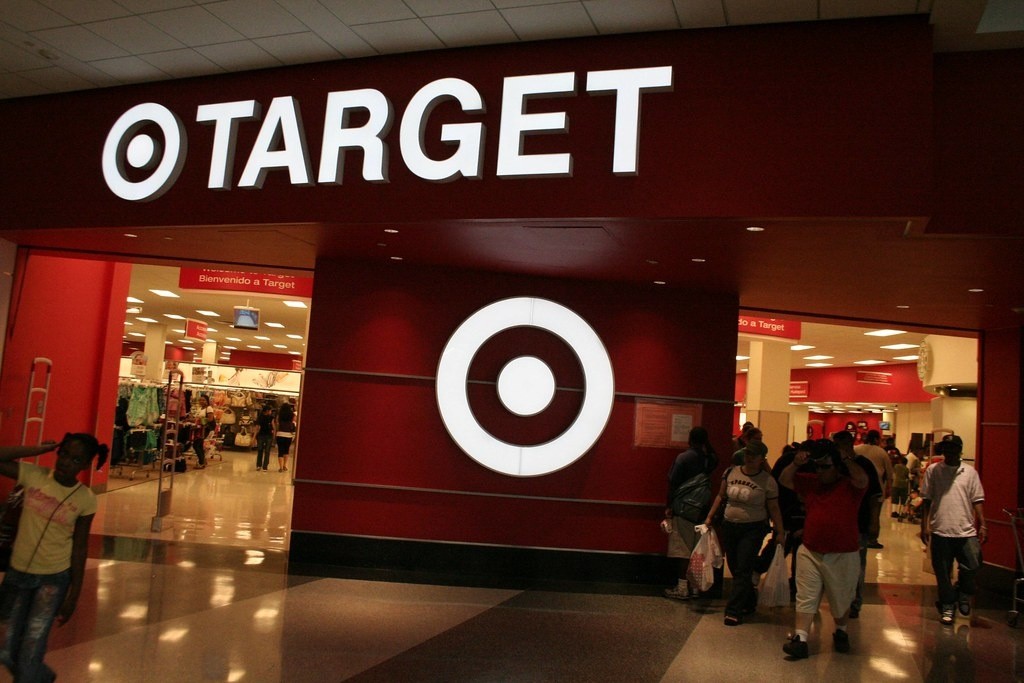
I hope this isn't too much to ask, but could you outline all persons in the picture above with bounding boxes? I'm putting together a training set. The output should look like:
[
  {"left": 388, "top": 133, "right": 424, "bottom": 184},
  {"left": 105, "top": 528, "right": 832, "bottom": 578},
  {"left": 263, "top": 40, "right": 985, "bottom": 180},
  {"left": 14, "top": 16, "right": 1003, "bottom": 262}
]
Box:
[
  {"left": 178, "top": 390, "right": 214, "bottom": 469},
  {"left": 665, "top": 421, "right": 944, "bottom": 661},
  {"left": 920, "top": 435, "right": 989, "bottom": 624},
  {"left": 0, "top": 432, "right": 109, "bottom": 683},
  {"left": 253, "top": 403, "right": 294, "bottom": 472}
]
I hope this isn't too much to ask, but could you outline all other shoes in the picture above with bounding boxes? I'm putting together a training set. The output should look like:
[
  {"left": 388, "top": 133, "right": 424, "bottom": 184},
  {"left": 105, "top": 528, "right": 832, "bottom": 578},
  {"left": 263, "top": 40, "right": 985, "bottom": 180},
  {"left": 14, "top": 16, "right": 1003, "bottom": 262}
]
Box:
[
  {"left": 832, "top": 628, "right": 850, "bottom": 653},
  {"left": 257, "top": 466, "right": 267, "bottom": 472},
  {"left": 959, "top": 592, "right": 970, "bottom": 616},
  {"left": 724, "top": 613, "right": 739, "bottom": 625},
  {"left": 866, "top": 542, "right": 883, "bottom": 549},
  {"left": 849, "top": 607, "right": 859, "bottom": 618},
  {"left": 782, "top": 634, "right": 808, "bottom": 658},
  {"left": 940, "top": 609, "right": 954, "bottom": 624},
  {"left": 278, "top": 466, "right": 287, "bottom": 472},
  {"left": 193, "top": 464, "right": 205, "bottom": 469}
]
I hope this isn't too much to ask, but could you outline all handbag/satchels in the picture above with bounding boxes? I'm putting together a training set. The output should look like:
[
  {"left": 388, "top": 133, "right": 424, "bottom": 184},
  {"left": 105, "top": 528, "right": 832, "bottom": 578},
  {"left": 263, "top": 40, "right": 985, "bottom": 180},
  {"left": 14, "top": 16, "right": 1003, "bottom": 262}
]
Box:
[
  {"left": 717, "top": 497, "right": 726, "bottom": 518},
  {"left": 685, "top": 524, "right": 723, "bottom": 593},
  {"left": 759, "top": 542, "right": 790, "bottom": 608}
]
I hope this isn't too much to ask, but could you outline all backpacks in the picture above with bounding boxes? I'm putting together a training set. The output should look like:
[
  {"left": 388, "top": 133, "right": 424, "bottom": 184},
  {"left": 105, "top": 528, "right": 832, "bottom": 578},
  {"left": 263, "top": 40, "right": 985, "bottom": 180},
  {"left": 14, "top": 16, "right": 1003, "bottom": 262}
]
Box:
[{"left": 671, "top": 454, "right": 711, "bottom": 523}]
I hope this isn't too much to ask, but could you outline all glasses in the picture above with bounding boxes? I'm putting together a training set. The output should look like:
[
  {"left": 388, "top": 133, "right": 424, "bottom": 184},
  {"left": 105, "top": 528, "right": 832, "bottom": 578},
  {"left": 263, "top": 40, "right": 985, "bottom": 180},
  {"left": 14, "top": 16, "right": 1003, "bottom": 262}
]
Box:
[{"left": 812, "top": 463, "right": 835, "bottom": 469}]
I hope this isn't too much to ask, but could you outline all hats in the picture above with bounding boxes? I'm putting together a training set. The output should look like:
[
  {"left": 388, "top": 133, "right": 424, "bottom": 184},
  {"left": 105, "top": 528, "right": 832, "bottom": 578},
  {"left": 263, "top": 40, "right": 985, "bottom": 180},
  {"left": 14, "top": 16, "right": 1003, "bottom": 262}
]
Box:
[
  {"left": 807, "top": 438, "right": 835, "bottom": 461},
  {"left": 939, "top": 434, "right": 962, "bottom": 446},
  {"left": 743, "top": 441, "right": 768, "bottom": 456}
]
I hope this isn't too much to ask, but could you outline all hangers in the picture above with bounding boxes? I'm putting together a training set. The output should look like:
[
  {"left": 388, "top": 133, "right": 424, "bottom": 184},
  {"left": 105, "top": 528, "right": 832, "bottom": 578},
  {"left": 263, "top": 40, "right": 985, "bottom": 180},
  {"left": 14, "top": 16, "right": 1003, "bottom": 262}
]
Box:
[{"left": 113, "top": 377, "right": 228, "bottom": 435}]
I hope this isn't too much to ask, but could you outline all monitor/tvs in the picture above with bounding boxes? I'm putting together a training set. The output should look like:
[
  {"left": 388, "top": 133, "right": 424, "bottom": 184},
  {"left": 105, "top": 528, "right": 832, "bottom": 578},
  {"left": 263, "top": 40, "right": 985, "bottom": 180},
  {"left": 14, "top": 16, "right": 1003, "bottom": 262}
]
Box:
[{"left": 233, "top": 308, "right": 260, "bottom": 331}]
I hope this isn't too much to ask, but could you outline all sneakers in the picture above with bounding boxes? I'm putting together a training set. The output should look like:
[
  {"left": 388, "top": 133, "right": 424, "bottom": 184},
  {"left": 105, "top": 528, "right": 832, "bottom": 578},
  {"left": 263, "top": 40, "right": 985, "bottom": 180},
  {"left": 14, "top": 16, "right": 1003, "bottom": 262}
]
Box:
[{"left": 664, "top": 585, "right": 689, "bottom": 599}]
